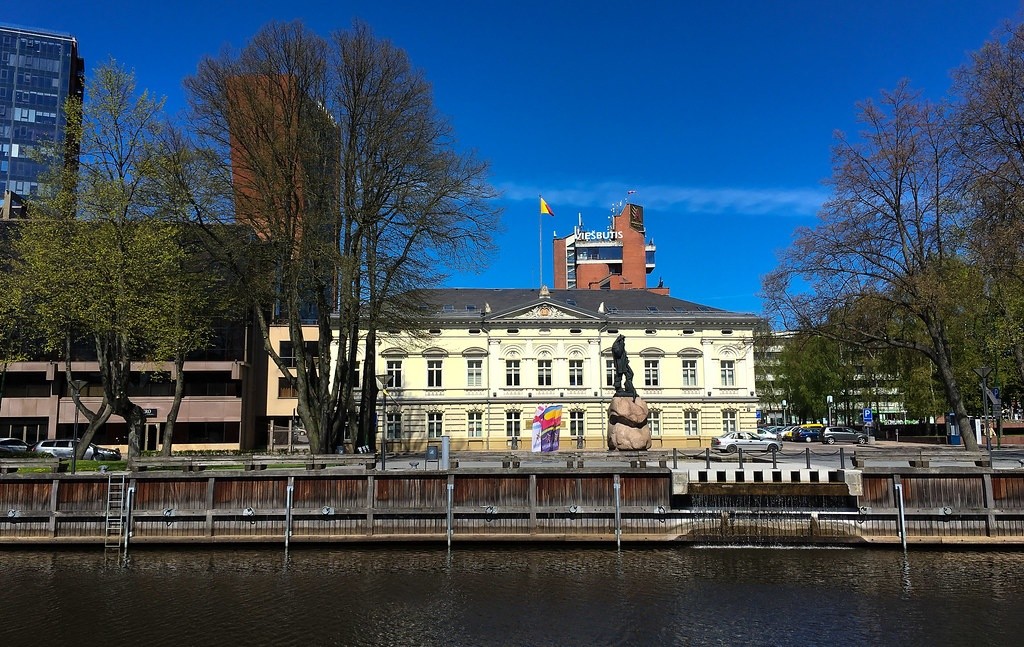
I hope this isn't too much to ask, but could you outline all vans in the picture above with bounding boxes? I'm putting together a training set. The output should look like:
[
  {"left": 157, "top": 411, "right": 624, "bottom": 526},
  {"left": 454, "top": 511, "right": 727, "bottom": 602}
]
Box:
[{"left": 787, "top": 423, "right": 823, "bottom": 441}]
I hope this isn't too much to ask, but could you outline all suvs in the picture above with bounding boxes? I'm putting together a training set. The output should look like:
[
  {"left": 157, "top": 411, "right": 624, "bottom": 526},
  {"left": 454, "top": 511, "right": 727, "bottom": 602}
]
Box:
[
  {"left": 821, "top": 426, "right": 867, "bottom": 445},
  {"left": 34, "top": 439, "right": 122, "bottom": 462}
]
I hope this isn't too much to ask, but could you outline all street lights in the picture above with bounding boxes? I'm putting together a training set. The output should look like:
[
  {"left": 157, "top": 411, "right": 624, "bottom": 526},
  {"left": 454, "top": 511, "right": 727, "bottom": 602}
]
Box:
[
  {"left": 971, "top": 366, "right": 993, "bottom": 468},
  {"left": 68, "top": 379, "right": 91, "bottom": 471},
  {"left": 375, "top": 374, "right": 396, "bottom": 469},
  {"left": 782, "top": 400, "right": 787, "bottom": 427},
  {"left": 826, "top": 395, "right": 833, "bottom": 427}
]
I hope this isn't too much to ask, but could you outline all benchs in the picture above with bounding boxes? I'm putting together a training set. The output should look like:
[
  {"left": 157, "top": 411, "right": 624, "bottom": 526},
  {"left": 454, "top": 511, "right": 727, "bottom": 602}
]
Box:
[
  {"left": 850, "top": 451, "right": 989, "bottom": 468},
  {"left": 0, "top": 458, "right": 69, "bottom": 473},
  {"left": 448, "top": 450, "right": 669, "bottom": 469},
  {"left": 129, "top": 454, "right": 376, "bottom": 473}
]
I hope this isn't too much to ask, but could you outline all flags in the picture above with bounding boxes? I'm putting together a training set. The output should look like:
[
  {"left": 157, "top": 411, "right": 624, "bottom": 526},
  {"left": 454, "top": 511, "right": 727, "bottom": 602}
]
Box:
[{"left": 540, "top": 198, "right": 555, "bottom": 216}]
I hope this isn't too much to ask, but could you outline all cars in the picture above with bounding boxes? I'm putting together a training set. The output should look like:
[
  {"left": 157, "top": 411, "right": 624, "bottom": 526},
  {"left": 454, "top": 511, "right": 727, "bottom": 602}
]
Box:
[
  {"left": 711, "top": 430, "right": 783, "bottom": 453},
  {"left": 764, "top": 425, "right": 799, "bottom": 441},
  {"left": 795, "top": 427, "right": 821, "bottom": 443},
  {"left": 758, "top": 428, "right": 777, "bottom": 441},
  {"left": 0, "top": 437, "right": 34, "bottom": 455}
]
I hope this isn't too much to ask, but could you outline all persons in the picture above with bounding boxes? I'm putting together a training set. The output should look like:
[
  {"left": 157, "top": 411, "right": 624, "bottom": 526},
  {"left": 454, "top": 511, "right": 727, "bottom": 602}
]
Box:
[
  {"left": 535, "top": 432, "right": 558, "bottom": 444},
  {"left": 983, "top": 425, "right": 996, "bottom": 450},
  {"left": 612, "top": 334, "right": 636, "bottom": 392},
  {"left": 758, "top": 419, "right": 766, "bottom": 433}
]
